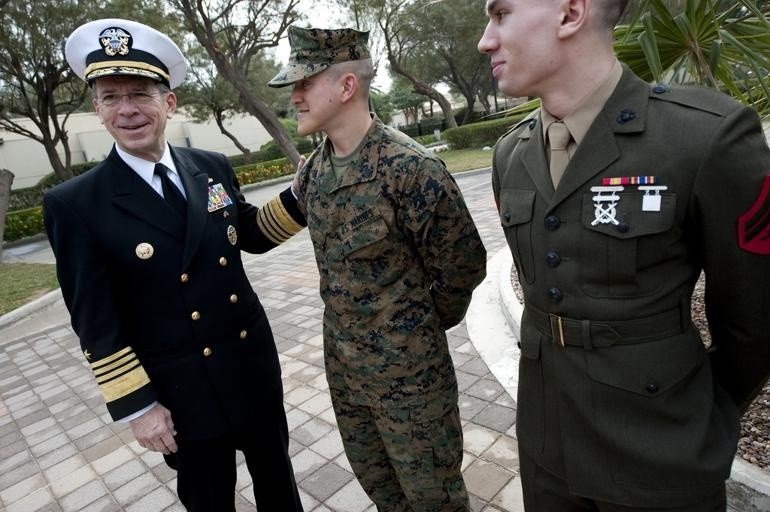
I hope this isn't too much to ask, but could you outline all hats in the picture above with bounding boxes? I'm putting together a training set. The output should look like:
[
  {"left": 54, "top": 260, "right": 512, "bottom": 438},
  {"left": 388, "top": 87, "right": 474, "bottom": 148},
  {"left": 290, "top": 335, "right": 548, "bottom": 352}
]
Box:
[
  {"left": 268, "top": 25, "right": 372, "bottom": 88},
  {"left": 65, "top": 18, "right": 188, "bottom": 90}
]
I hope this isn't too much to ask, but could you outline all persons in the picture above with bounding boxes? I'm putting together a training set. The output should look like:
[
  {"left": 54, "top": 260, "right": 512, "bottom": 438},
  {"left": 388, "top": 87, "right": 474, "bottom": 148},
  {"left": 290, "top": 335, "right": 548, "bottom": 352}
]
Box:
[
  {"left": 266, "top": 22, "right": 490, "bottom": 510},
  {"left": 39, "top": 19, "right": 321, "bottom": 512},
  {"left": 473, "top": 2, "right": 770, "bottom": 511}
]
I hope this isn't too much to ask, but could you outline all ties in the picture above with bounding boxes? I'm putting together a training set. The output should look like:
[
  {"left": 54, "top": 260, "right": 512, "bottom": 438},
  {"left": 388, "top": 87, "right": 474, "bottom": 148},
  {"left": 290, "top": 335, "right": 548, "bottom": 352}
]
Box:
[
  {"left": 154, "top": 164, "right": 188, "bottom": 213},
  {"left": 548, "top": 121, "right": 570, "bottom": 194}
]
